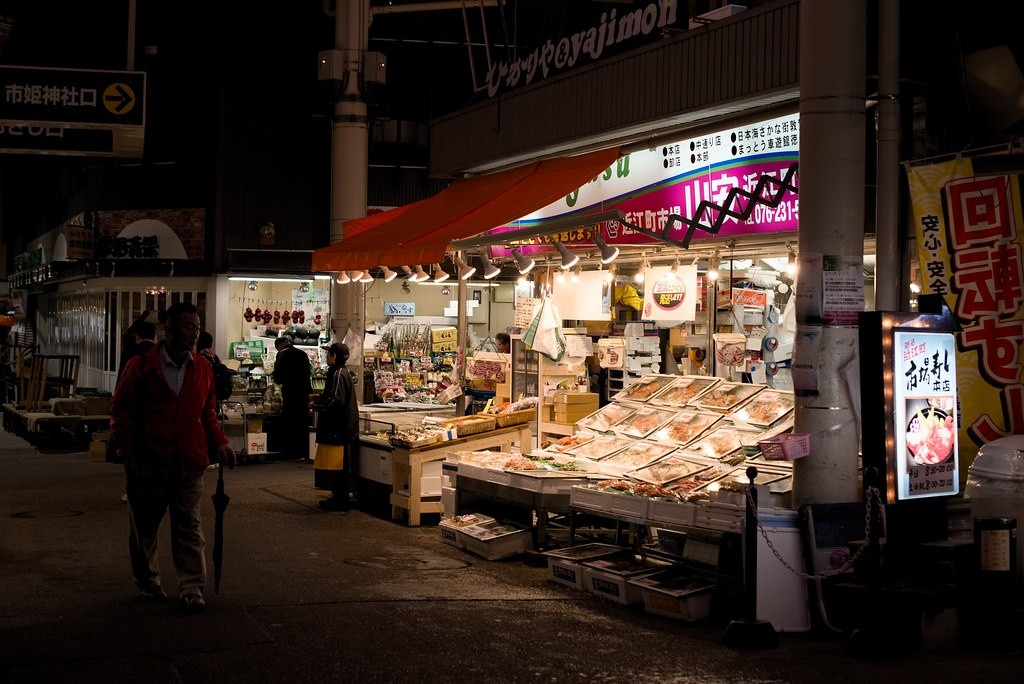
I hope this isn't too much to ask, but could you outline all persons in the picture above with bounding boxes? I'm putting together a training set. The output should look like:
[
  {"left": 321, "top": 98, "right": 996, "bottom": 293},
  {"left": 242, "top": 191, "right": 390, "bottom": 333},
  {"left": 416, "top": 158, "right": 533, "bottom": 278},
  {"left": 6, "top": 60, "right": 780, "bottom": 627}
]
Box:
[
  {"left": 116, "top": 321, "right": 156, "bottom": 502},
  {"left": 495, "top": 332, "right": 510, "bottom": 354},
  {"left": 196, "top": 331, "right": 221, "bottom": 470},
  {"left": 273, "top": 337, "right": 312, "bottom": 461},
  {"left": 108, "top": 303, "right": 236, "bottom": 606},
  {"left": 310, "top": 342, "right": 360, "bottom": 512}
]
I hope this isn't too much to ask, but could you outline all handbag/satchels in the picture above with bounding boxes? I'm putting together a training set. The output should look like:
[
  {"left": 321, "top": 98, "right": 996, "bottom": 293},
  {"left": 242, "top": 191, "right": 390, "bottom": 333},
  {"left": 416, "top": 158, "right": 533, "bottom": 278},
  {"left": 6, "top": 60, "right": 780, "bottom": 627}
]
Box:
[{"left": 106, "top": 437, "right": 125, "bottom": 465}]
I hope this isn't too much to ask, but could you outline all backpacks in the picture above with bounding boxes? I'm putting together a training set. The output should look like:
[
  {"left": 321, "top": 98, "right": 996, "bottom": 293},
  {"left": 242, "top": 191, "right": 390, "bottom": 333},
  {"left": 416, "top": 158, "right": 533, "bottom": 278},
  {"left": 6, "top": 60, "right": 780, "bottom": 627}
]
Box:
[{"left": 201, "top": 353, "right": 233, "bottom": 401}]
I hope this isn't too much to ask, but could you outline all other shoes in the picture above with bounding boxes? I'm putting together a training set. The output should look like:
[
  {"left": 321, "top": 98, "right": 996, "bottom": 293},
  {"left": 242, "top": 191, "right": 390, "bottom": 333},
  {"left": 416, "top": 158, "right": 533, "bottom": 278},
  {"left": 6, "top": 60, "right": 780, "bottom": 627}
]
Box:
[
  {"left": 205, "top": 463, "right": 220, "bottom": 470},
  {"left": 319, "top": 499, "right": 349, "bottom": 511},
  {"left": 288, "top": 457, "right": 305, "bottom": 461}
]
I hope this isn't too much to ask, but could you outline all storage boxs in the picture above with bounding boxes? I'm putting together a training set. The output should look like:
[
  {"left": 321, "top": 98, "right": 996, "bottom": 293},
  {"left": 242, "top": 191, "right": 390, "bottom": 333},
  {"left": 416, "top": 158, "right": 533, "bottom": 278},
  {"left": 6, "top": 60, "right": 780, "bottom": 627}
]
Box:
[
  {"left": 219, "top": 339, "right": 327, "bottom": 458},
  {"left": 364, "top": 328, "right": 795, "bottom": 621},
  {"left": 89, "top": 429, "right": 112, "bottom": 461}
]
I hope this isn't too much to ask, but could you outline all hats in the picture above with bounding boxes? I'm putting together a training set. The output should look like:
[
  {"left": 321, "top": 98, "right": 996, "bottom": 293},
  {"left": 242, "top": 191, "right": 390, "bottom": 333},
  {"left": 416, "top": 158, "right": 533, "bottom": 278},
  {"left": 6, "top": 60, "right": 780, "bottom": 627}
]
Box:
[{"left": 321, "top": 343, "right": 349, "bottom": 360}]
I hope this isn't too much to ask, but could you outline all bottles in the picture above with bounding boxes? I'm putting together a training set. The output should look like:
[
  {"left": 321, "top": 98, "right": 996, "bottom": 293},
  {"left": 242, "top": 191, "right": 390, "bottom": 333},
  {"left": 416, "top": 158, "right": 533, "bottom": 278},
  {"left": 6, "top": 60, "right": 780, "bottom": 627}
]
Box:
[{"left": 515, "top": 348, "right": 538, "bottom": 400}]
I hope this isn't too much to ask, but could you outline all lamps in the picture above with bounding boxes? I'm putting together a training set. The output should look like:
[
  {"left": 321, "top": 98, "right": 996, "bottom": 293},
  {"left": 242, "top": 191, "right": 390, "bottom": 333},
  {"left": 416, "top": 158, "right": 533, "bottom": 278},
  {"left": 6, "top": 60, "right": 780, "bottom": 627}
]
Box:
[
  {"left": 455, "top": 256, "right": 475, "bottom": 279},
  {"left": 911, "top": 280, "right": 923, "bottom": 293},
  {"left": 401, "top": 282, "right": 409, "bottom": 294},
  {"left": 481, "top": 252, "right": 501, "bottom": 279},
  {"left": 434, "top": 263, "right": 449, "bottom": 282},
  {"left": 227, "top": 272, "right": 332, "bottom": 292},
  {"left": 512, "top": 233, "right": 797, "bottom": 293},
  {"left": 337, "top": 271, "right": 350, "bottom": 283},
  {"left": 359, "top": 269, "right": 374, "bottom": 283},
  {"left": 441, "top": 284, "right": 451, "bottom": 294},
  {"left": 349, "top": 270, "right": 363, "bottom": 283},
  {"left": 416, "top": 265, "right": 429, "bottom": 281},
  {"left": 379, "top": 266, "right": 398, "bottom": 283},
  {"left": 400, "top": 265, "right": 417, "bottom": 282}
]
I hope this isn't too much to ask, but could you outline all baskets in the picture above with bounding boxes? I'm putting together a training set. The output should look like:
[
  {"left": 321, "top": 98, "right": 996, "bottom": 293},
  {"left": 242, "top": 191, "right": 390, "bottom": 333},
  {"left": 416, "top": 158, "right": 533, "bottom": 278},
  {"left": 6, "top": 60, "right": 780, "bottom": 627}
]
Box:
[
  {"left": 758, "top": 434, "right": 810, "bottom": 460},
  {"left": 436, "top": 385, "right": 462, "bottom": 404},
  {"left": 477, "top": 403, "right": 536, "bottom": 427},
  {"left": 436, "top": 415, "right": 496, "bottom": 435}
]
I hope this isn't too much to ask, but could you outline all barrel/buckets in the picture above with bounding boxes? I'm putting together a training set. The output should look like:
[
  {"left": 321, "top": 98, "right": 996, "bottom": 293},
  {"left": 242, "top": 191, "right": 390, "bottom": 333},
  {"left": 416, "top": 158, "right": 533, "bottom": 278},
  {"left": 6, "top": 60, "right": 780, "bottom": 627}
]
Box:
[{"left": 598, "top": 321, "right": 623, "bottom": 368}]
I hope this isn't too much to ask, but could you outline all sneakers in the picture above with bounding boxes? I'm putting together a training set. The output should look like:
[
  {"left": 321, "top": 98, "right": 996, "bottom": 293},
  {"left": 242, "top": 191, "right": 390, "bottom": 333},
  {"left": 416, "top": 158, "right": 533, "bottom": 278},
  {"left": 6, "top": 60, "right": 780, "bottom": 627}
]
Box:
[
  {"left": 135, "top": 579, "right": 168, "bottom": 602},
  {"left": 178, "top": 580, "right": 206, "bottom": 612}
]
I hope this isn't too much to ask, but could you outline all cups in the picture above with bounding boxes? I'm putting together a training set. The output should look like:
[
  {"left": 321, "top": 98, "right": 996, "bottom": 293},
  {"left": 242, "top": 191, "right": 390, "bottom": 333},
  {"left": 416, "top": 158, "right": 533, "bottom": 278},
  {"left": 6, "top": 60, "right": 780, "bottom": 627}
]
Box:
[{"left": 578, "top": 385, "right": 588, "bottom": 392}]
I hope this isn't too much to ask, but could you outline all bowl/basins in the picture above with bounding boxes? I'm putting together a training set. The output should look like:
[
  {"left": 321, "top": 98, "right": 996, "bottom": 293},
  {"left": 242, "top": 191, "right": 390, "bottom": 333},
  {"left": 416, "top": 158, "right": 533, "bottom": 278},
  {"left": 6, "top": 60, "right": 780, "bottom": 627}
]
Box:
[{"left": 905, "top": 408, "right": 953, "bottom": 465}]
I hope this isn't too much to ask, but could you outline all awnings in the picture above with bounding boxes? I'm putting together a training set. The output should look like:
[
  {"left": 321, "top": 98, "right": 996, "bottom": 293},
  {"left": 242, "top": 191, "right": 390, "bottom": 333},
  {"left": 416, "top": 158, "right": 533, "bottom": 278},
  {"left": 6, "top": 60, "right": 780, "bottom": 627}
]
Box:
[{"left": 310, "top": 151, "right": 621, "bottom": 274}]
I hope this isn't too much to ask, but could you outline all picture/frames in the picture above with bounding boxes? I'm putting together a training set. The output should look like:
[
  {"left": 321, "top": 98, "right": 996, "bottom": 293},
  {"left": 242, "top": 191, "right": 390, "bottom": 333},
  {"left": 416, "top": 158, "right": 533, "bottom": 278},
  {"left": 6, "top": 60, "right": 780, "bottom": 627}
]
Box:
[{"left": 474, "top": 289, "right": 481, "bottom": 303}]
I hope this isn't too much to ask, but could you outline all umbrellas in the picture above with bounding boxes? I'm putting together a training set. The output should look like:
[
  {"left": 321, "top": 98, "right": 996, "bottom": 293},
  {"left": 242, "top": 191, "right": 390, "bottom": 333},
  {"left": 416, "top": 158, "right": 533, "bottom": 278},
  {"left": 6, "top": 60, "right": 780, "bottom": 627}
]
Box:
[{"left": 212, "top": 453, "right": 235, "bottom": 595}]
